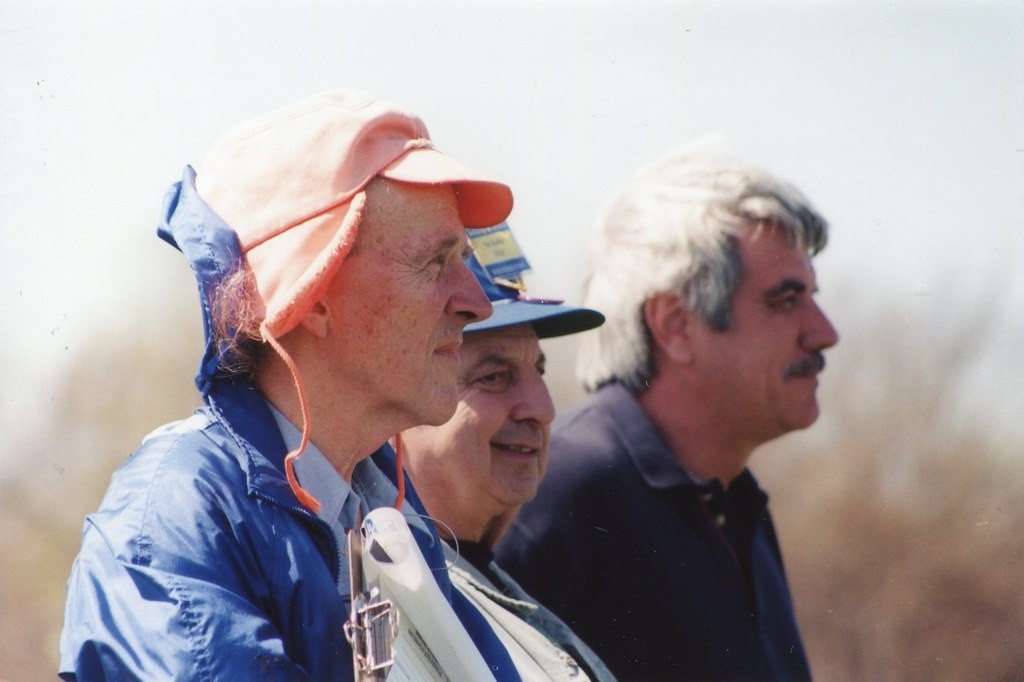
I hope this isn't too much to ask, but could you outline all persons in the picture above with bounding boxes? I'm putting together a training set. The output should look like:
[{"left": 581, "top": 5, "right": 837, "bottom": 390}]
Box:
[
  {"left": 388, "top": 219, "right": 619, "bottom": 682},
  {"left": 56, "top": 92, "right": 515, "bottom": 682},
  {"left": 491, "top": 159, "right": 838, "bottom": 682}
]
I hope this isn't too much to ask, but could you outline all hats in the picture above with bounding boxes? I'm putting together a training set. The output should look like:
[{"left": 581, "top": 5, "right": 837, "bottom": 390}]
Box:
[
  {"left": 174, "top": 99, "right": 515, "bottom": 510},
  {"left": 463, "top": 222, "right": 606, "bottom": 340}
]
